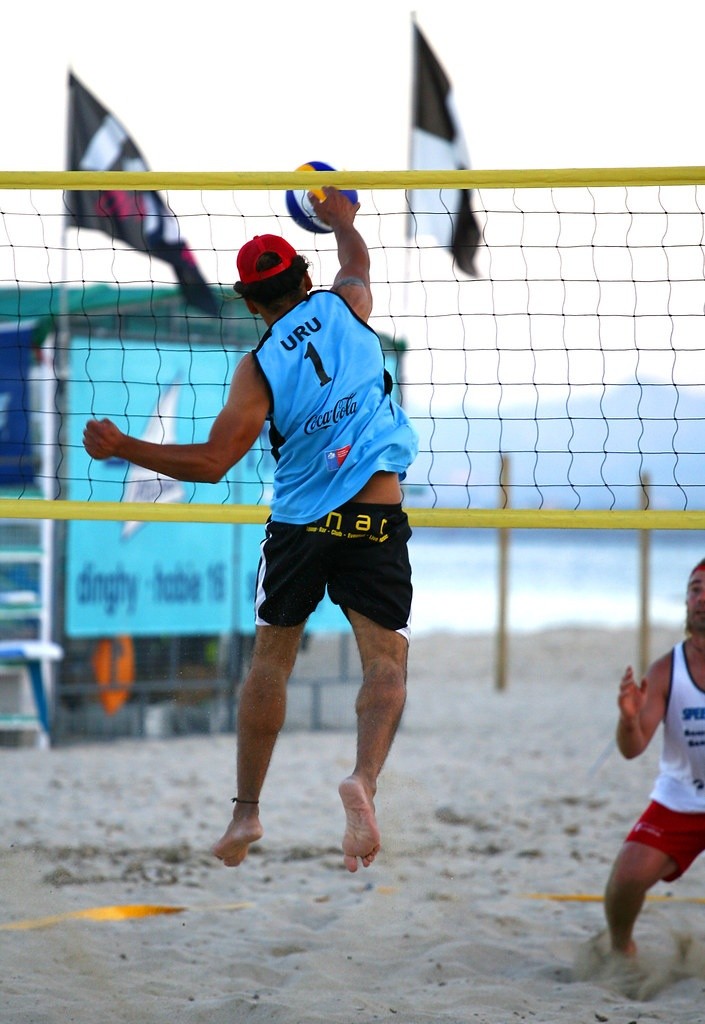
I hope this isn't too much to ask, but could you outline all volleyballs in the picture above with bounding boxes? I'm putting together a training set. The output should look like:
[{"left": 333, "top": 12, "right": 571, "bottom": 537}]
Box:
[{"left": 283, "top": 160, "right": 361, "bottom": 236}]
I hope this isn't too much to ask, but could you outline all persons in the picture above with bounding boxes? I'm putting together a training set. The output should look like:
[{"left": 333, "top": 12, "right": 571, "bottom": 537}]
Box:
[
  {"left": 604, "top": 558, "right": 705, "bottom": 954},
  {"left": 82, "top": 186, "right": 417, "bottom": 873}
]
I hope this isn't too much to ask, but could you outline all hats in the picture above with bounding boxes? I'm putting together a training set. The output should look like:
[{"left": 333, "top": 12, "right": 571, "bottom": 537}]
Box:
[{"left": 237, "top": 233, "right": 298, "bottom": 284}]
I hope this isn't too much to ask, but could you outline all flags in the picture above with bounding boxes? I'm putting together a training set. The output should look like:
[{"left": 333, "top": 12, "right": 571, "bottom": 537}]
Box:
[
  {"left": 60, "top": 76, "right": 221, "bottom": 316},
  {"left": 409, "top": 33, "right": 483, "bottom": 280}
]
